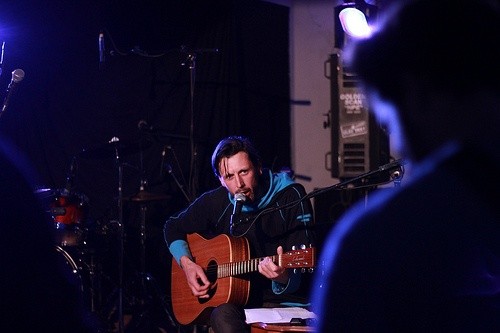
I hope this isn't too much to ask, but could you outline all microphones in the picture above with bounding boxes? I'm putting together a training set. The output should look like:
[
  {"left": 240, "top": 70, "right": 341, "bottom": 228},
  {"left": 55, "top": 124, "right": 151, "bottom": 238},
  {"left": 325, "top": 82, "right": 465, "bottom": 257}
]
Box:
[
  {"left": 229, "top": 191, "right": 247, "bottom": 236},
  {"left": 1, "top": 69, "right": 25, "bottom": 114}
]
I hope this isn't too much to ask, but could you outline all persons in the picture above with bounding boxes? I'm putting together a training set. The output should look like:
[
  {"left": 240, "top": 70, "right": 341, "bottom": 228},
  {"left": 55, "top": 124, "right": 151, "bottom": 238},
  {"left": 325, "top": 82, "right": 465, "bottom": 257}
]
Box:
[
  {"left": 312, "top": 0, "right": 500, "bottom": 333},
  {"left": 163, "top": 136, "right": 314, "bottom": 333},
  {"left": 0, "top": 153, "right": 99, "bottom": 333}
]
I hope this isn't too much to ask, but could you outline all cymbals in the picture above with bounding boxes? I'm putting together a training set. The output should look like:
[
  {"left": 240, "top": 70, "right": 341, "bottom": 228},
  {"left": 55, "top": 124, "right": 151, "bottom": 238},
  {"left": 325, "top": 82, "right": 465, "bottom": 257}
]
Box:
[{"left": 119, "top": 190, "right": 173, "bottom": 203}]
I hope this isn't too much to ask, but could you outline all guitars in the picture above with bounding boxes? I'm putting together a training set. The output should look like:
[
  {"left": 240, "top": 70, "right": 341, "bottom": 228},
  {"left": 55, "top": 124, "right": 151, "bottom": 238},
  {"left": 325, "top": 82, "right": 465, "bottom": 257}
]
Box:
[{"left": 170, "top": 229, "right": 318, "bottom": 326}]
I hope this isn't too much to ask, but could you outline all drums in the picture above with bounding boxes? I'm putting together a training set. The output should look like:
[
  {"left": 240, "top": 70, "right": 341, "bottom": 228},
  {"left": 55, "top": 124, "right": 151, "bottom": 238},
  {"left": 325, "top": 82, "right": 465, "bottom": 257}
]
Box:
[{"left": 33, "top": 187, "right": 92, "bottom": 230}]
